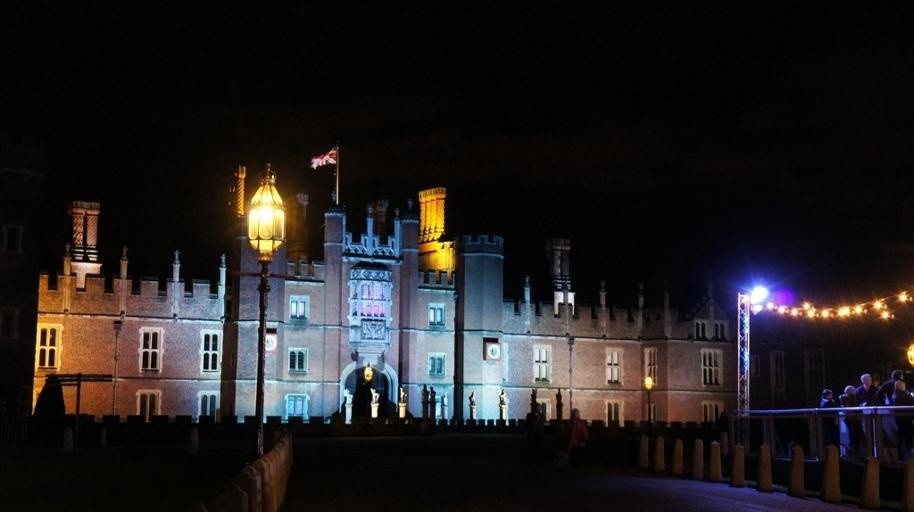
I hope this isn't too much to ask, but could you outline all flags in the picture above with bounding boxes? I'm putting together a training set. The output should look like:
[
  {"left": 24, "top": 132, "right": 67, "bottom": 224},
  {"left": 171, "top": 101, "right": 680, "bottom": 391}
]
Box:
[{"left": 310, "top": 146, "right": 336, "bottom": 170}]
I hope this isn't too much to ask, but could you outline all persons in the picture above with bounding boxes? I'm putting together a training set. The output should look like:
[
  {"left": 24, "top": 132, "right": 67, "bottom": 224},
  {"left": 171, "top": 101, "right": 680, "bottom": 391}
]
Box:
[
  {"left": 525, "top": 401, "right": 546, "bottom": 472},
  {"left": 565, "top": 407, "right": 587, "bottom": 469},
  {"left": 816, "top": 368, "right": 914, "bottom": 466}
]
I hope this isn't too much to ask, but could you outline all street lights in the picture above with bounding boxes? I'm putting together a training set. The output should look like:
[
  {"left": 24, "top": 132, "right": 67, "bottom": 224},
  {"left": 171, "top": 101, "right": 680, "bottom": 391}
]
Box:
[
  {"left": 113, "top": 319, "right": 122, "bottom": 415},
  {"left": 567, "top": 335, "right": 575, "bottom": 409},
  {"left": 247, "top": 160, "right": 289, "bottom": 458},
  {"left": 644, "top": 376, "right": 653, "bottom": 423}
]
